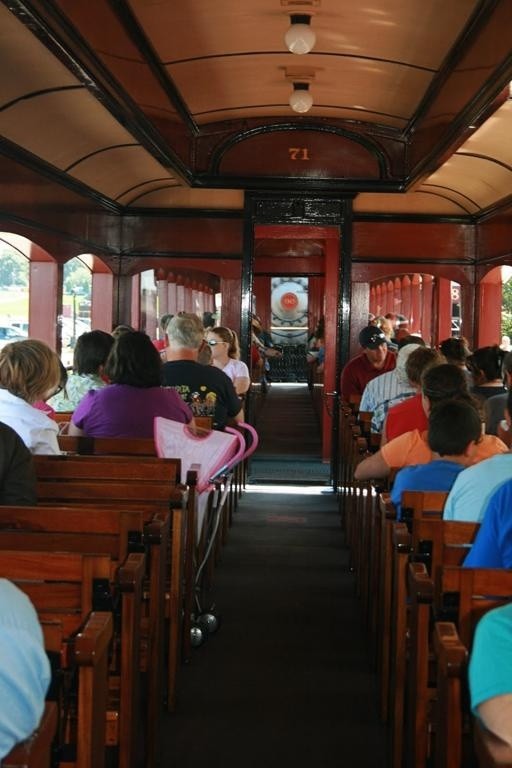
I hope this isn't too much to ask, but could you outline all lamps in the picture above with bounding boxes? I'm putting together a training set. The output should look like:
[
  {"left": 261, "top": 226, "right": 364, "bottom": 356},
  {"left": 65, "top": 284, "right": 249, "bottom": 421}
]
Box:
[{"left": 280, "top": 1, "right": 318, "bottom": 113}]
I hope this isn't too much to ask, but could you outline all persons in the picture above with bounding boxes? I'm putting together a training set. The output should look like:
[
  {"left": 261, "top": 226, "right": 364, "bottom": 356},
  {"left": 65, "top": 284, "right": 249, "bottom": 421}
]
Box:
[
  {"left": 31, "top": 311, "right": 284, "bottom": 426},
  {"left": 304, "top": 308, "right": 511, "bottom": 451},
  {"left": 0, "top": 422, "right": 49, "bottom": 506},
  {"left": 351, "top": 364, "right": 511, "bottom": 482},
  {"left": 0, "top": 578, "right": 65, "bottom": 764},
  {"left": 389, "top": 399, "right": 481, "bottom": 549},
  {"left": 465, "top": 599, "right": 512, "bottom": 749},
  {"left": 455, "top": 481, "right": 511, "bottom": 602},
  {"left": 69, "top": 329, "right": 199, "bottom": 440},
  {"left": 442, "top": 454, "right": 512, "bottom": 523},
  {"left": 0, "top": 338, "right": 67, "bottom": 456}
]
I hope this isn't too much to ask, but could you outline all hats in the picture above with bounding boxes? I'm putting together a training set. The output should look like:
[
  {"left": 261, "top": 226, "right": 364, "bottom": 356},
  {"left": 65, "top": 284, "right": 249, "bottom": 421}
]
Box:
[{"left": 359, "top": 325, "right": 386, "bottom": 348}]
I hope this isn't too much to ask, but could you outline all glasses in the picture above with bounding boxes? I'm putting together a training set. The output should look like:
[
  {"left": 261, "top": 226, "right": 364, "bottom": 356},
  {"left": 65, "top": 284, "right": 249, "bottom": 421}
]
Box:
[
  {"left": 45, "top": 360, "right": 68, "bottom": 401},
  {"left": 208, "top": 340, "right": 226, "bottom": 345}
]
